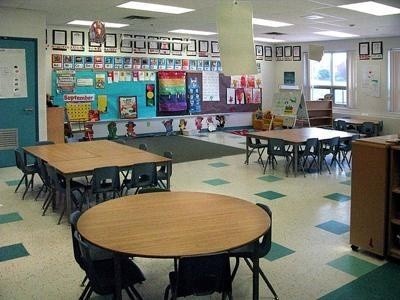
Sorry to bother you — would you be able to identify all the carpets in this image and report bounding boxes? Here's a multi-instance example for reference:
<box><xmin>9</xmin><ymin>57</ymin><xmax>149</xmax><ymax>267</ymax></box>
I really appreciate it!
<box><xmin>316</xmin><ymin>263</ymin><xmax>400</xmax><ymax>300</ymax></box>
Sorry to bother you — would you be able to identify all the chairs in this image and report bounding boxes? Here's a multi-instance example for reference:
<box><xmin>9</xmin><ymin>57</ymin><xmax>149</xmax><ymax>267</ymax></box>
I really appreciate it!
<box><xmin>243</xmin><ymin>117</ymin><xmax>384</xmax><ymax>177</ymax></box>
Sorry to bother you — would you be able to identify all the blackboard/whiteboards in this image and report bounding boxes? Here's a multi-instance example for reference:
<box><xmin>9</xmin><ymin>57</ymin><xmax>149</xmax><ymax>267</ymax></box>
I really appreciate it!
<box><xmin>51</xmin><ymin>71</ymin><xmax>263</xmax><ymax>124</ymax></box>
<box><xmin>272</xmin><ymin>91</ymin><xmax>302</xmax><ymax>116</ymax></box>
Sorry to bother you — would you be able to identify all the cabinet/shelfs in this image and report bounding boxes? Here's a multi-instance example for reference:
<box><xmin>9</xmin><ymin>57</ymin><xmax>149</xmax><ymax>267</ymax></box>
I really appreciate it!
<box><xmin>349</xmin><ymin>134</ymin><xmax>400</xmax><ymax>263</ymax></box>
<box><xmin>47</xmin><ymin>106</ymin><xmax>66</xmax><ymax>145</ymax></box>
<box><xmin>301</xmin><ymin>100</ymin><xmax>333</xmax><ymax>129</ymax></box>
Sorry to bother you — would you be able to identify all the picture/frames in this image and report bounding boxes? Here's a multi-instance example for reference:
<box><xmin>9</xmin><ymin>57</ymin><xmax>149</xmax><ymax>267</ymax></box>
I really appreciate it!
<box><xmin>372</xmin><ymin>41</ymin><xmax>382</xmax><ymax>54</ymax></box>
<box><xmin>51</xmin><ymin>29</ymin><xmax>302</xmax><ymax>57</ymax></box>
<box><xmin>119</xmin><ymin>95</ymin><xmax>138</xmax><ymax>119</ymax></box>
<box><xmin>358</xmin><ymin>42</ymin><xmax>369</xmax><ymax>55</ymax></box>
